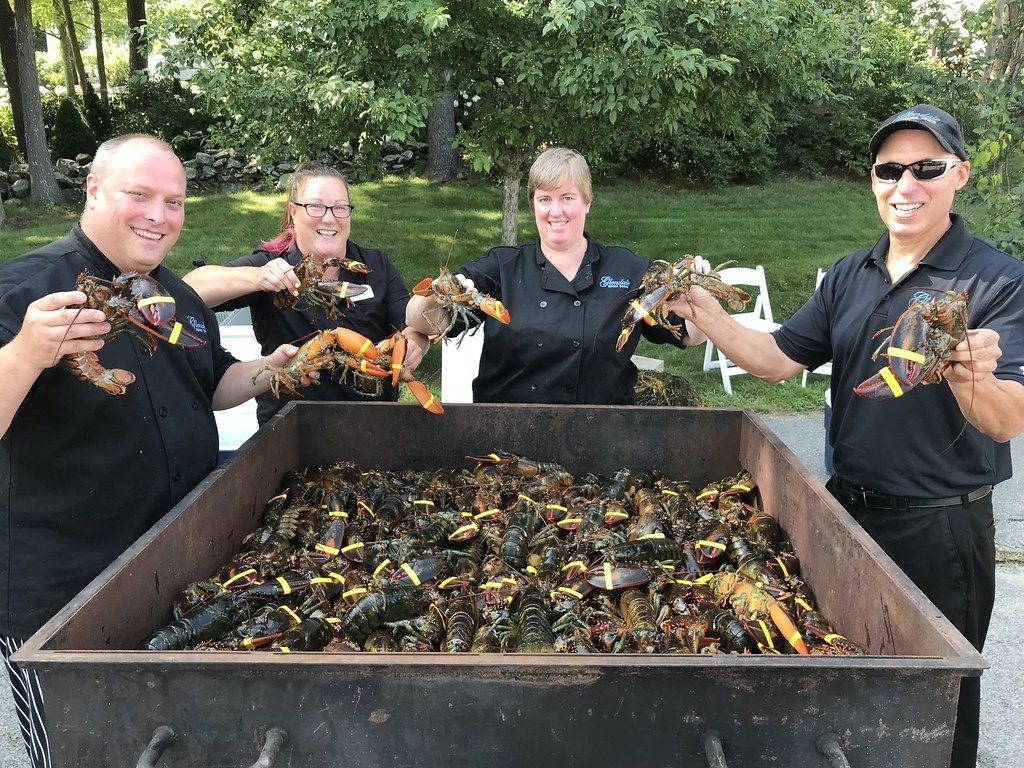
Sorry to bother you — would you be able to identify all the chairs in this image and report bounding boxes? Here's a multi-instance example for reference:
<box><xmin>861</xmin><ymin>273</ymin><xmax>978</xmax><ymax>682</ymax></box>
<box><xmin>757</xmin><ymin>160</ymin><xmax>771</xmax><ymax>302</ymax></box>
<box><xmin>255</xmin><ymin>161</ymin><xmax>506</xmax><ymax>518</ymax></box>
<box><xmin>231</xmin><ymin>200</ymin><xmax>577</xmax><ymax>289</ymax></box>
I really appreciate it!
<box><xmin>801</xmin><ymin>268</ymin><xmax>832</xmax><ymax>388</ymax></box>
<box><xmin>702</xmin><ymin>265</ymin><xmax>786</xmax><ymax>395</ymax></box>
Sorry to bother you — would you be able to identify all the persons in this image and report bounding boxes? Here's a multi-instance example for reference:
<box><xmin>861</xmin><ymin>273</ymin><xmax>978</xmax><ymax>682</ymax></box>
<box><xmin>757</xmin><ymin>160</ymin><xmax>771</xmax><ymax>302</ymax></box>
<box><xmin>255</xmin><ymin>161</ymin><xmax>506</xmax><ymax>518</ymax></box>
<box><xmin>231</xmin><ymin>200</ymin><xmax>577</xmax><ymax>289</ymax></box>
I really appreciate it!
<box><xmin>0</xmin><ymin>133</ymin><xmax>333</xmax><ymax>768</ymax></box>
<box><xmin>405</xmin><ymin>147</ymin><xmax>719</xmax><ymax>406</ymax></box>
<box><xmin>657</xmin><ymin>103</ymin><xmax>1024</xmax><ymax>768</ymax></box>
<box><xmin>181</xmin><ymin>160</ymin><xmax>429</xmax><ymax>430</ymax></box>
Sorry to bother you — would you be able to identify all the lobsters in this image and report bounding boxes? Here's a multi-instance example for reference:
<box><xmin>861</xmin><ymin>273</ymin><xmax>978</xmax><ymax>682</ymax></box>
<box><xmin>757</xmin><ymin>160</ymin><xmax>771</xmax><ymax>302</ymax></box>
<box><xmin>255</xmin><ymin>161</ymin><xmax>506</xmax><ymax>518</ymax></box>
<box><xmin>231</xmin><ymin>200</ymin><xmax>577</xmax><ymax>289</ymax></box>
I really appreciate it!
<box><xmin>60</xmin><ymin>268</ymin><xmax>210</xmax><ymax>397</ymax></box>
<box><xmin>251</xmin><ymin>327</ymin><xmax>444</xmax><ymax>415</ymax></box>
<box><xmin>844</xmin><ymin>287</ymin><xmax>975</xmax><ymax>456</ymax></box>
<box><xmin>411</xmin><ymin>228</ymin><xmax>511</xmax><ymax>349</ymax></box>
<box><xmin>270</xmin><ymin>253</ymin><xmax>373</xmax><ymax>324</ymax></box>
<box><xmin>142</xmin><ymin>446</ymin><xmax>866</xmax><ymax>655</ymax></box>
<box><xmin>616</xmin><ymin>254</ymin><xmax>752</xmax><ymax>355</ymax></box>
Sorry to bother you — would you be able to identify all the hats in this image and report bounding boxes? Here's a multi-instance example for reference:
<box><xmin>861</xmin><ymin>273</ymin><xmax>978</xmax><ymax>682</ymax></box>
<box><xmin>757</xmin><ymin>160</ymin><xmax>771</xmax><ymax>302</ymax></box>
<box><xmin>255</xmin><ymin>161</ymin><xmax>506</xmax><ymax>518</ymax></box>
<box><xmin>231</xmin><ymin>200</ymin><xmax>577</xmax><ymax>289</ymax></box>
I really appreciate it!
<box><xmin>870</xmin><ymin>104</ymin><xmax>966</xmax><ymax>160</ymax></box>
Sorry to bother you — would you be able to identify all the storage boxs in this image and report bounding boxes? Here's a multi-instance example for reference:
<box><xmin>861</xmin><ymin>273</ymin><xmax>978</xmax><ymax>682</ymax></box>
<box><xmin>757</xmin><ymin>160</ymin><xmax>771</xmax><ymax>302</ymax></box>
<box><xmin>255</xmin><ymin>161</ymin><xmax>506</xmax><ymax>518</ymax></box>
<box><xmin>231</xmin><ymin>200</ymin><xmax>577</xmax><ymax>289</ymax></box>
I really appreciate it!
<box><xmin>8</xmin><ymin>402</ymin><xmax>991</xmax><ymax>768</ymax></box>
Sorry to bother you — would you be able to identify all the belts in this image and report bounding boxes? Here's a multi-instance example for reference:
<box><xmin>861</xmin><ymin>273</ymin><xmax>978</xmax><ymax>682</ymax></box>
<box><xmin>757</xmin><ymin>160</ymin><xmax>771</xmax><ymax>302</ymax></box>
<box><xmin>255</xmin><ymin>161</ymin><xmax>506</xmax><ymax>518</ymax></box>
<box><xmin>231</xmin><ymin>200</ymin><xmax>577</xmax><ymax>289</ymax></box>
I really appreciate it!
<box><xmin>835</xmin><ymin>476</ymin><xmax>992</xmax><ymax>510</ymax></box>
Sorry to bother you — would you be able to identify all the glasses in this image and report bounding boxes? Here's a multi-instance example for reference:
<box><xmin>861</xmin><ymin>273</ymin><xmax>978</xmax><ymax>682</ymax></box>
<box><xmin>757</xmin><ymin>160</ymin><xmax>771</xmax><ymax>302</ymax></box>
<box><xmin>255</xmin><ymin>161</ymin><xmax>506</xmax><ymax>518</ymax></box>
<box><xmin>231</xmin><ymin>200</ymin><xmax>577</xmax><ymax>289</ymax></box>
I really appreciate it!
<box><xmin>873</xmin><ymin>158</ymin><xmax>961</xmax><ymax>182</ymax></box>
<box><xmin>291</xmin><ymin>201</ymin><xmax>354</xmax><ymax>218</ymax></box>
